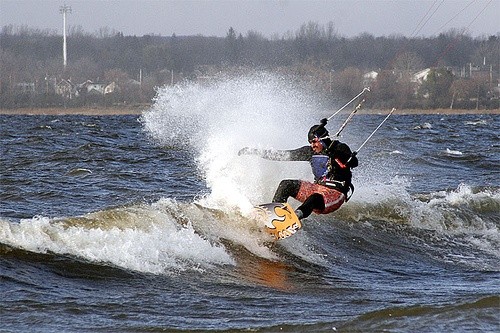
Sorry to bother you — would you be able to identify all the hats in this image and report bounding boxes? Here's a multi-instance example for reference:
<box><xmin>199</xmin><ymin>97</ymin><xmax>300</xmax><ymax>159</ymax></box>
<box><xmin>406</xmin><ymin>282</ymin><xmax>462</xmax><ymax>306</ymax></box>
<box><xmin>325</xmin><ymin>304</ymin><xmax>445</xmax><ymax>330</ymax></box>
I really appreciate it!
<box><xmin>308</xmin><ymin>125</ymin><xmax>332</xmax><ymax>148</ymax></box>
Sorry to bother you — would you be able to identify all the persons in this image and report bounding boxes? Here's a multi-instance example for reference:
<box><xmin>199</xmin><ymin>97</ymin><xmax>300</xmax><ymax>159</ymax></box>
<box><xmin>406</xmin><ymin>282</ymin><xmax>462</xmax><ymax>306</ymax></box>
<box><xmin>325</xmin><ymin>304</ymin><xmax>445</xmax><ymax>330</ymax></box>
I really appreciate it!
<box><xmin>238</xmin><ymin>125</ymin><xmax>359</xmax><ymax>220</ymax></box>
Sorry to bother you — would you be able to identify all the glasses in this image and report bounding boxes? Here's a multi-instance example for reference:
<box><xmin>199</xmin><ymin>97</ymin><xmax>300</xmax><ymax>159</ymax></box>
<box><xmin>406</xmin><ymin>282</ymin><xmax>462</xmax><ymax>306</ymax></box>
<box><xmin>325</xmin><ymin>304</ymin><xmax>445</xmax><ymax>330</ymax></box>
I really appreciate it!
<box><xmin>308</xmin><ymin>131</ymin><xmax>327</xmax><ymax>144</ymax></box>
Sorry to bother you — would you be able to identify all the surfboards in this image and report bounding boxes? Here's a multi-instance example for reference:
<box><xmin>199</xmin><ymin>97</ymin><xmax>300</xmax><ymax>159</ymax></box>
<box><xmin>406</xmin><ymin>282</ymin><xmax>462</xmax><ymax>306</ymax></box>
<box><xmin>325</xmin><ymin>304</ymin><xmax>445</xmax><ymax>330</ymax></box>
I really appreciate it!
<box><xmin>254</xmin><ymin>203</ymin><xmax>302</xmax><ymax>240</ymax></box>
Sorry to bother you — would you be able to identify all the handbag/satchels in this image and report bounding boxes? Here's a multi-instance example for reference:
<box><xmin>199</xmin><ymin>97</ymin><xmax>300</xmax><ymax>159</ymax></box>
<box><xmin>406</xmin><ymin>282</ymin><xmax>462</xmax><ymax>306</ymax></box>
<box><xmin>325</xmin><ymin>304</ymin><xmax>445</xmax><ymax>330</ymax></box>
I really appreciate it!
<box><xmin>342</xmin><ymin>181</ymin><xmax>354</xmax><ymax>202</ymax></box>
